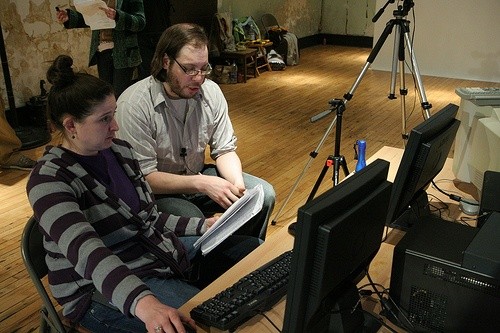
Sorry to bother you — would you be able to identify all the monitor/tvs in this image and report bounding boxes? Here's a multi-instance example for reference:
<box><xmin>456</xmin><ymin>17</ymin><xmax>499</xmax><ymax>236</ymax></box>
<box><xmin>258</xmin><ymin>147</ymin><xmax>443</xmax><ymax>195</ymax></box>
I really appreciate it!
<box><xmin>283</xmin><ymin>158</ymin><xmax>394</xmax><ymax>333</ymax></box>
<box><xmin>387</xmin><ymin>103</ymin><xmax>462</xmax><ymax>231</ymax></box>
<box><xmin>452</xmin><ymin>97</ymin><xmax>500</xmax><ymax>191</ymax></box>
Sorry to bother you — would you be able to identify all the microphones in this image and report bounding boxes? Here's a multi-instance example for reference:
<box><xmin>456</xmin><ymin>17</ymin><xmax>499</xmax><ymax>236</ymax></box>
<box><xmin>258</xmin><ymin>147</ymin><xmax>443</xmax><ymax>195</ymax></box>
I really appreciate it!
<box><xmin>180</xmin><ymin>148</ymin><xmax>187</xmax><ymax>161</ymax></box>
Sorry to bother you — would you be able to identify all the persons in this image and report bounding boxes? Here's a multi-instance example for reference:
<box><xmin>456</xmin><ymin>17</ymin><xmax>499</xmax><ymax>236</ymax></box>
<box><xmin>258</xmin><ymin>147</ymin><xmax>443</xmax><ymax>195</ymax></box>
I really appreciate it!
<box><xmin>0</xmin><ymin>93</ymin><xmax>37</xmax><ymax>170</ymax></box>
<box><xmin>26</xmin><ymin>55</ymin><xmax>265</xmax><ymax>333</ymax></box>
<box><xmin>112</xmin><ymin>24</ymin><xmax>275</xmax><ymax>240</ymax></box>
<box><xmin>56</xmin><ymin>0</ymin><xmax>146</xmax><ymax>100</ymax></box>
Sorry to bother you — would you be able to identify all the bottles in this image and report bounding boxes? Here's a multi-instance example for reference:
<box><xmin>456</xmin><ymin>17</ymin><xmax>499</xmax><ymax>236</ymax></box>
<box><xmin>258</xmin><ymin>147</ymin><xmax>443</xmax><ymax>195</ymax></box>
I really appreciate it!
<box><xmin>354</xmin><ymin>140</ymin><xmax>367</xmax><ymax>175</ymax></box>
<box><xmin>249</xmin><ymin>25</ymin><xmax>255</xmax><ymax>41</ymax></box>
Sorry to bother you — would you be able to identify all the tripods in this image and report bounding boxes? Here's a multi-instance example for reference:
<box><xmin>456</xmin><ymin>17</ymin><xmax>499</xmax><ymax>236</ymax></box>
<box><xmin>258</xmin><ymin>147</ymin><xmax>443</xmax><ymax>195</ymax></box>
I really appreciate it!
<box><xmin>271</xmin><ymin>0</ymin><xmax>431</xmax><ymax>226</ymax></box>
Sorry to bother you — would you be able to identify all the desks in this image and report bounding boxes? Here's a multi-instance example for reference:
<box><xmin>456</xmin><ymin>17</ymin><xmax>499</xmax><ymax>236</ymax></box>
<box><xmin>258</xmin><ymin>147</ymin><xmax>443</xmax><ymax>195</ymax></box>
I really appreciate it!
<box><xmin>153</xmin><ymin>146</ymin><xmax>480</xmax><ymax>333</ymax></box>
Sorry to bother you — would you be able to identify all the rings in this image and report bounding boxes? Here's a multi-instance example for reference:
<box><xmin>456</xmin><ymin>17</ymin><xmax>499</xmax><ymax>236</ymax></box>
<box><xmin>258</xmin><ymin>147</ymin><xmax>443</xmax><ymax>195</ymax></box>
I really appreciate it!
<box><xmin>155</xmin><ymin>327</ymin><xmax>162</xmax><ymax>333</ymax></box>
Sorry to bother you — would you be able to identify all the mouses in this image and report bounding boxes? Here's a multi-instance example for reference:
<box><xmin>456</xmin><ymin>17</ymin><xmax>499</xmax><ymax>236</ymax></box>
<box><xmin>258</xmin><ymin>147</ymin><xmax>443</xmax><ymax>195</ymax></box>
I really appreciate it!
<box><xmin>171</xmin><ymin>322</ymin><xmax>197</xmax><ymax>333</ymax></box>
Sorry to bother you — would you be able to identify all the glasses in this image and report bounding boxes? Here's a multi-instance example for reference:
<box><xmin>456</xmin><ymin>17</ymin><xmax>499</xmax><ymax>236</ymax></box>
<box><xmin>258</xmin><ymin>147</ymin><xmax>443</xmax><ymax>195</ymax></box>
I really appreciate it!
<box><xmin>174</xmin><ymin>57</ymin><xmax>213</xmax><ymax>76</ymax></box>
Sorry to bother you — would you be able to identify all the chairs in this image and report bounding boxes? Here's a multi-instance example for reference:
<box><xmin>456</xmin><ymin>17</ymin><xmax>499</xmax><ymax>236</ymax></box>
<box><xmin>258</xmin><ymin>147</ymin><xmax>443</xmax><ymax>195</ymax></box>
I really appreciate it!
<box><xmin>213</xmin><ymin>12</ymin><xmax>258</xmax><ymax>83</ymax></box>
<box><xmin>262</xmin><ymin>14</ymin><xmax>300</xmax><ymax>65</ymax></box>
<box><xmin>234</xmin><ymin>17</ymin><xmax>273</xmax><ymax>75</ymax></box>
<box><xmin>21</xmin><ymin>214</ymin><xmax>90</xmax><ymax>333</ymax></box>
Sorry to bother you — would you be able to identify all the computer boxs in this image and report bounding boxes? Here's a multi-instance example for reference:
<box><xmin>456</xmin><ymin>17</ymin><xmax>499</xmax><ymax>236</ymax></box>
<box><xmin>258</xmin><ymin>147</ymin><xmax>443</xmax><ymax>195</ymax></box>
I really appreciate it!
<box><xmin>384</xmin><ymin>214</ymin><xmax>500</xmax><ymax>333</ymax></box>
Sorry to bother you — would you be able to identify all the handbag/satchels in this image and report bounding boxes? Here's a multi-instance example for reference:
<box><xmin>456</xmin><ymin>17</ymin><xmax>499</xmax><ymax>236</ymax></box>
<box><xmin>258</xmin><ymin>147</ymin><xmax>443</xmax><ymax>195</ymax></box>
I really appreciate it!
<box><xmin>224</xmin><ymin>35</ymin><xmax>237</xmax><ymax>51</ymax></box>
<box><xmin>213</xmin><ymin>63</ymin><xmax>237</xmax><ymax>84</ymax></box>
<box><xmin>5</xmin><ymin>103</ymin><xmax>52</xmax><ymax>153</ymax></box>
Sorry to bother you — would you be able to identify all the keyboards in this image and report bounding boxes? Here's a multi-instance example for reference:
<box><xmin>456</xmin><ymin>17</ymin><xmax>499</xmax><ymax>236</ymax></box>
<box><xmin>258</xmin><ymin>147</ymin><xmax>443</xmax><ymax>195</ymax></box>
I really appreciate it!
<box><xmin>455</xmin><ymin>87</ymin><xmax>500</xmax><ymax>106</ymax></box>
<box><xmin>190</xmin><ymin>248</ymin><xmax>294</xmax><ymax>333</ymax></box>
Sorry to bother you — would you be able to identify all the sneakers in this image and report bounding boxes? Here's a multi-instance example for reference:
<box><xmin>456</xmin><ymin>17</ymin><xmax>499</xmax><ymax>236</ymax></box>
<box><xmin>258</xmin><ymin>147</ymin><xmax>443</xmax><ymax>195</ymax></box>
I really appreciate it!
<box><xmin>2</xmin><ymin>152</ymin><xmax>38</xmax><ymax>170</ymax></box>
